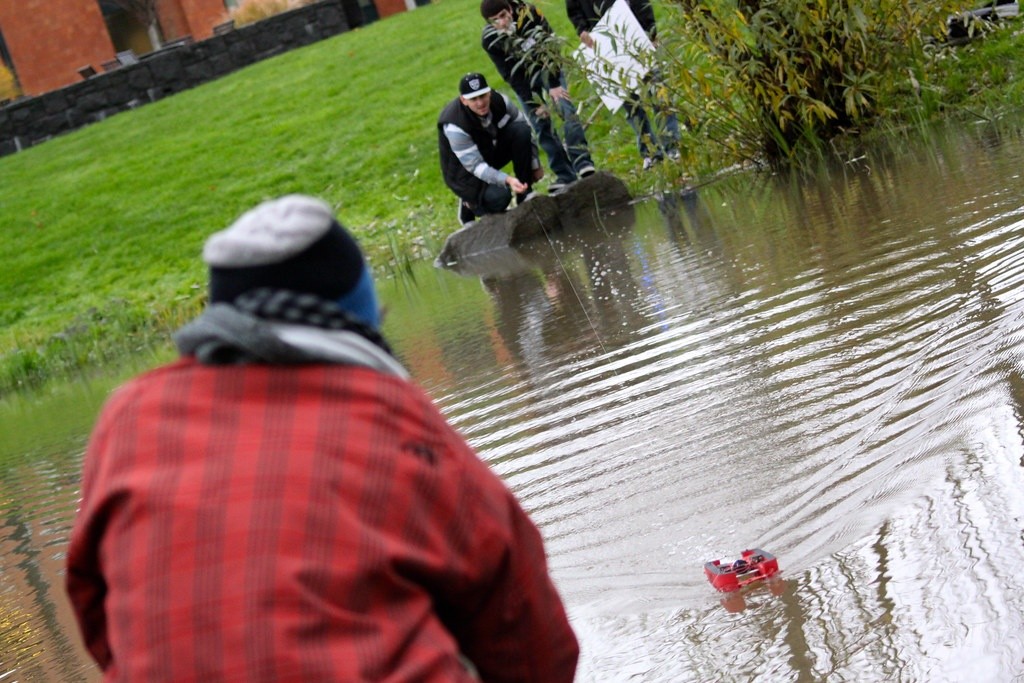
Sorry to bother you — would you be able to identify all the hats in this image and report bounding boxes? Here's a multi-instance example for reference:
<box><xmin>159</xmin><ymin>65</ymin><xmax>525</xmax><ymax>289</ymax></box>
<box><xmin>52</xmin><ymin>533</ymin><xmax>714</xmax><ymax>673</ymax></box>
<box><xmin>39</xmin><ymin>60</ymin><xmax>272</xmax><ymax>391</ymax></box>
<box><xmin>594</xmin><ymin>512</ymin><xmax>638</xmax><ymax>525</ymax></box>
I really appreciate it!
<box><xmin>202</xmin><ymin>194</ymin><xmax>379</xmax><ymax>332</ymax></box>
<box><xmin>460</xmin><ymin>73</ymin><xmax>491</xmax><ymax>100</ymax></box>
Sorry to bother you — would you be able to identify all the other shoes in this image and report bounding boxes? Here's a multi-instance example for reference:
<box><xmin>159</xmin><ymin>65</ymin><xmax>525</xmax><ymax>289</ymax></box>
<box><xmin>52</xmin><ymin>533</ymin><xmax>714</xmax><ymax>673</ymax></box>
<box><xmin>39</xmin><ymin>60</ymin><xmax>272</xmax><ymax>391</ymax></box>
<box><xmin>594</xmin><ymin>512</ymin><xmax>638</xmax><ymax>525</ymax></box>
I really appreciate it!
<box><xmin>643</xmin><ymin>158</ymin><xmax>663</xmax><ymax>171</ymax></box>
<box><xmin>577</xmin><ymin>160</ymin><xmax>596</xmax><ymax>178</ymax></box>
<box><xmin>547</xmin><ymin>175</ymin><xmax>579</xmax><ymax>194</ymax></box>
<box><xmin>457</xmin><ymin>198</ymin><xmax>476</xmax><ymax>227</ymax></box>
<box><xmin>667</xmin><ymin>149</ymin><xmax>681</xmax><ymax>159</ymax></box>
<box><xmin>516</xmin><ymin>188</ymin><xmax>538</xmax><ymax>207</ymax></box>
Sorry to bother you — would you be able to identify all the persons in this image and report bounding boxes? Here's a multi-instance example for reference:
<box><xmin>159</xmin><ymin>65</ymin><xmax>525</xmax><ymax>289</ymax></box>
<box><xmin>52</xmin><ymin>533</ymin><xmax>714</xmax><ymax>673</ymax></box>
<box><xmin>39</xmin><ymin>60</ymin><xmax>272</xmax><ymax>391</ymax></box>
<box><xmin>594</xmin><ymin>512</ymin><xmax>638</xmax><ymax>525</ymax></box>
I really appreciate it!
<box><xmin>437</xmin><ymin>72</ymin><xmax>544</xmax><ymax>226</ymax></box>
<box><xmin>481</xmin><ymin>0</ymin><xmax>597</xmax><ymax>191</ymax></box>
<box><xmin>64</xmin><ymin>193</ymin><xmax>578</xmax><ymax>683</ymax></box>
<box><xmin>565</xmin><ymin>0</ymin><xmax>683</xmax><ymax>174</ymax></box>
